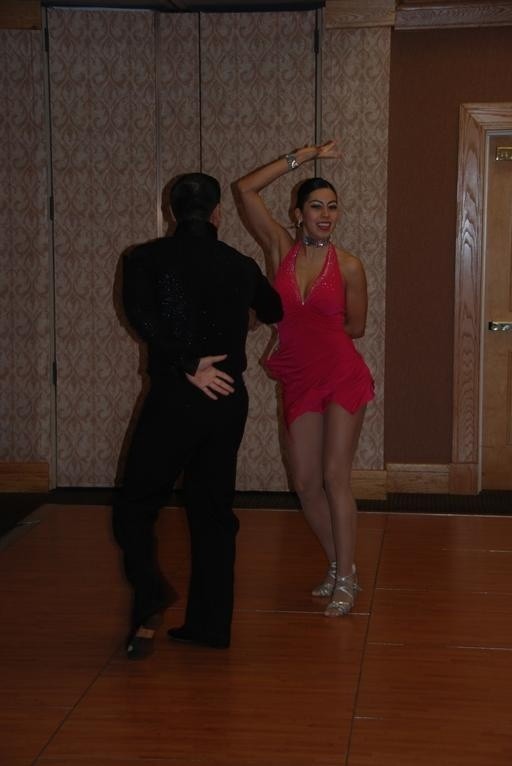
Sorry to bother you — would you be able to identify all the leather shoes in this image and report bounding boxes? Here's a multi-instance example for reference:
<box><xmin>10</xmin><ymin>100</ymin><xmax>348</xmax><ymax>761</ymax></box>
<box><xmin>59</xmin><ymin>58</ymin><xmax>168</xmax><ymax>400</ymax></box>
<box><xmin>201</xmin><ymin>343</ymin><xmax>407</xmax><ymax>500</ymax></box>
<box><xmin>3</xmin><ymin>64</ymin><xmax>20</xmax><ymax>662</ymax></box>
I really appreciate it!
<box><xmin>167</xmin><ymin>622</ymin><xmax>231</xmax><ymax>649</ymax></box>
<box><xmin>125</xmin><ymin>588</ymin><xmax>179</xmax><ymax>662</ymax></box>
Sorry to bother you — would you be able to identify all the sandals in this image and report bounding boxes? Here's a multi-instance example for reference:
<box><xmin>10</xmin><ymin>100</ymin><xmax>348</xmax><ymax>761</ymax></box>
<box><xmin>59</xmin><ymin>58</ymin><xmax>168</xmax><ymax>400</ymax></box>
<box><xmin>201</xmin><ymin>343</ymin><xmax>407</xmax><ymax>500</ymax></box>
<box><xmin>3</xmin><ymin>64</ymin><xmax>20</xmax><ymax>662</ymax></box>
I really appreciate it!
<box><xmin>323</xmin><ymin>562</ymin><xmax>362</xmax><ymax>618</ymax></box>
<box><xmin>312</xmin><ymin>561</ymin><xmax>338</xmax><ymax>597</ymax></box>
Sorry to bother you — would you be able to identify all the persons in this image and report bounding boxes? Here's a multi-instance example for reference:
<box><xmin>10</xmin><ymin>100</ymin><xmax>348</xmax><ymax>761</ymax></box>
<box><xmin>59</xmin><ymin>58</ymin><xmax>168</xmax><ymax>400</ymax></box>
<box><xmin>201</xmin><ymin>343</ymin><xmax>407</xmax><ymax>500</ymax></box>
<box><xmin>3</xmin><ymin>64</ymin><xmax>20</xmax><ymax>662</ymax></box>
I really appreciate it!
<box><xmin>108</xmin><ymin>169</ymin><xmax>284</xmax><ymax>659</ymax></box>
<box><xmin>236</xmin><ymin>142</ymin><xmax>379</xmax><ymax>620</ymax></box>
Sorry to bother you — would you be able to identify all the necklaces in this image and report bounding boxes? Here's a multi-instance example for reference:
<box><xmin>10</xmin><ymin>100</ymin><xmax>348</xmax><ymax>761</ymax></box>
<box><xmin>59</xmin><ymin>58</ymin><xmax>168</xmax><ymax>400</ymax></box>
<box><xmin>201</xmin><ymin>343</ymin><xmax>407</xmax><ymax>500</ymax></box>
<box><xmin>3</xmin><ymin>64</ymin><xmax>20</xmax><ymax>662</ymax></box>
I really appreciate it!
<box><xmin>301</xmin><ymin>234</ymin><xmax>331</xmax><ymax>248</ymax></box>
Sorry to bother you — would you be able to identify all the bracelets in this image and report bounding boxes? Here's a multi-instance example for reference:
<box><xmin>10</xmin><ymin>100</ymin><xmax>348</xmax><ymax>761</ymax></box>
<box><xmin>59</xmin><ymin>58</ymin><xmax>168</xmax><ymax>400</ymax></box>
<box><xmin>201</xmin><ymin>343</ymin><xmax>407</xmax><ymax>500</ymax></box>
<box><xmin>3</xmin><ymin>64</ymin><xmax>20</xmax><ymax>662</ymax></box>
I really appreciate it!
<box><xmin>279</xmin><ymin>152</ymin><xmax>299</xmax><ymax>170</ymax></box>
